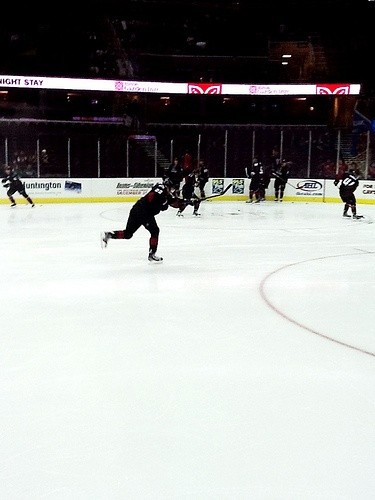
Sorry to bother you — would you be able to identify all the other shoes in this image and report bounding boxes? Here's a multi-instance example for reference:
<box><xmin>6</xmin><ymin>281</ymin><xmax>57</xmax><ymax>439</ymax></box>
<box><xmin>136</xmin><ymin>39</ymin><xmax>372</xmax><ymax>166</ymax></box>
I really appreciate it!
<box><xmin>353</xmin><ymin>213</ymin><xmax>363</xmax><ymax>220</ymax></box>
<box><xmin>11</xmin><ymin>204</ymin><xmax>16</xmax><ymax>207</ymax></box>
<box><xmin>246</xmin><ymin>200</ymin><xmax>253</xmax><ymax>203</ymax></box>
<box><xmin>280</xmin><ymin>198</ymin><xmax>283</xmax><ymax>202</ymax></box>
<box><xmin>274</xmin><ymin>198</ymin><xmax>278</xmax><ymax>201</ymax></box>
<box><xmin>342</xmin><ymin>213</ymin><xmax>351</xmax><ymax>217</ymax></box>
<box><xmin>31</xmin><ymin>204</ymin><xmax>35</xmax><ymax>208</ymax></box>
<box><xmin>254</xmin><ymin>199</ymin><xmax>262</xmax><ymax>204</ymax></box>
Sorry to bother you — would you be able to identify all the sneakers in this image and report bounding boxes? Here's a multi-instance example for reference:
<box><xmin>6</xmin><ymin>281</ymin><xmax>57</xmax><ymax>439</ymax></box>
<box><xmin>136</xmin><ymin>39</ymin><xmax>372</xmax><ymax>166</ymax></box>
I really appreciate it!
<box><xmin>192</xmin><ymin>210</ymin><xmax>201</xmax><ymax>216</ymax></box>
<box><xmin>101</xmin><ymin>231</ymin><xmax>110</xmax><ymax>247</ymax></box>
<box><xmin>148</xmin><ymin>254</ymin><xmax>163</xmax><ymax>263</ymax></box>
<box><xmin>176</xmin><ymin>211</ymin><xmax>184</xmax><ymax>218</ymax></box>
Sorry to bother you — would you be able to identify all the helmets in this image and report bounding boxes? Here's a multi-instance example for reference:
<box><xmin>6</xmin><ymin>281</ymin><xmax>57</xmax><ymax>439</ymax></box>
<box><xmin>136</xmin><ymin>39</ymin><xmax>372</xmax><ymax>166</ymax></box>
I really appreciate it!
<box><xmin>5</xmin><ymin>166</ymin><xmax>11</xmax><ymax>175</ymax></box>
<box><xmin>193</xmin><ymin>169</ymin><xmax>200</xmax><ymax>175</ymax></box>
<box><xmin>253</xmin><ymin>159</ymin><xmax>259</xmax><ymax>167</ymax></box>
<box><xmin>281</xmin><ymin>159</ymin><xmax>287</xmax><ymax>166</ymax></box>
<box><xmin>164</xmin><ymin>178</ymin><xmax>174</xmax><ymax>188</ymax></box>
<box><xmin>352</xmin><ymin>170</ymin><xmax>358</xmax><ymax>178</ymax></box>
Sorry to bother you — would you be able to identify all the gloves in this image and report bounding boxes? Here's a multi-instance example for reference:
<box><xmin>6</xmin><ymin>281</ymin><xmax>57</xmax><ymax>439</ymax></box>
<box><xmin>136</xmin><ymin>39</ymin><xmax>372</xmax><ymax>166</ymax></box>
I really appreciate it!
<box><xmin>334</xmin><ymin>181</ymin><xmax>339</xmax><ymax>186</ymax></box>
<box><xmin>190</xmin><ymin>198</ymin><xmax>198</xmax><ymax>206</ymax></box>
<box><xmin>1</xmin><ymin>179</ymin><xmax>6</xmax><ymax>183</ymax></box>
<box><xmin>3</xmin><ymin>183</ymin><xmax>9</xmax><ymax>188</ymax></box>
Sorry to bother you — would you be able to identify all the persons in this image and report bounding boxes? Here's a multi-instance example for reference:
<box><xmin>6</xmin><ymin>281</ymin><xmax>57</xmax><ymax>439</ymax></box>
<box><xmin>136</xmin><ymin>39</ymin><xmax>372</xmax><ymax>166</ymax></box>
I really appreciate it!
<box><xmin>163</xmin><ymin>145</ymin><xmax>292</xmax><ymax>218</ymax></box>
<box><xmin>0</xmin><ymin>145</ymin><xmax>50</xmax><ymax>208</ymax></box>
<box><xmin>101</xmin><ymin>178</ymin><xmax>198</xmax><ymax>264</ymax></box>
<box><xmin>318</xmin><ymin>160</ymin><xmax>375</xmax><ymax>219</ymax></box>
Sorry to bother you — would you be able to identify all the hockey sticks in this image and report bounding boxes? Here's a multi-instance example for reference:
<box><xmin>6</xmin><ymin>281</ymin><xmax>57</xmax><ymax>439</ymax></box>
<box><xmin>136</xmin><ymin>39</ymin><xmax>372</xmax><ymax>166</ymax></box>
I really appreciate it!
<box><xmin>279</xmin><ymin>176</ymin><xmax>304</xmax><ymax>189</ymax></box>
<box><xmin>196</xmin><ymin>183</ymin><xmax>233</xmax><ymax>200</ymax></box>
